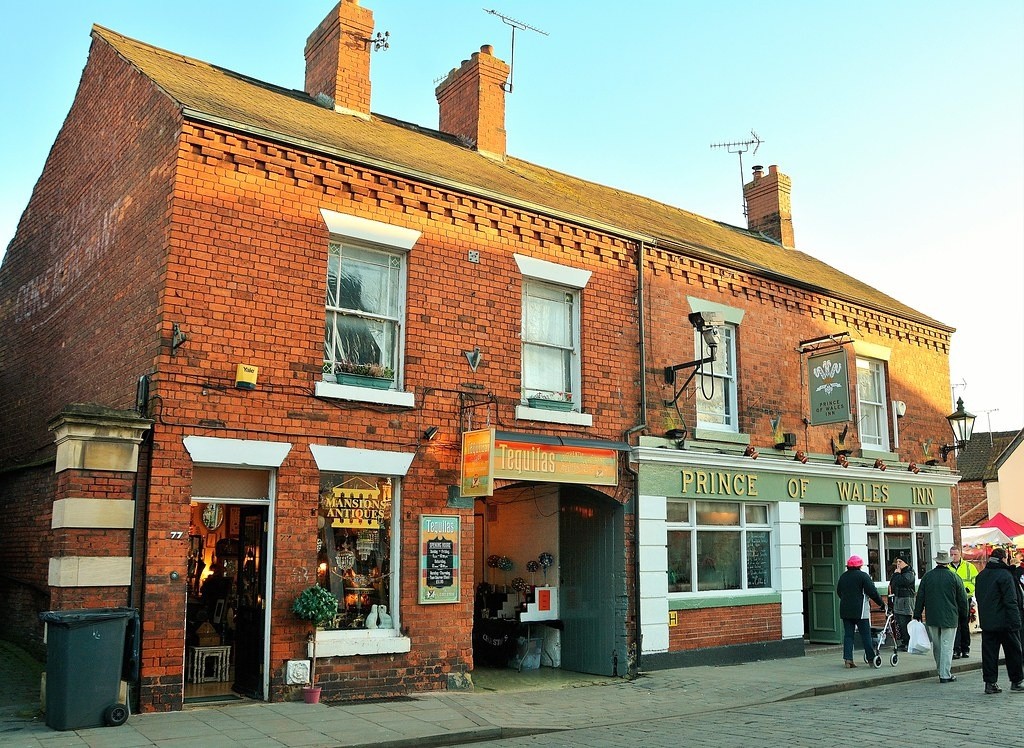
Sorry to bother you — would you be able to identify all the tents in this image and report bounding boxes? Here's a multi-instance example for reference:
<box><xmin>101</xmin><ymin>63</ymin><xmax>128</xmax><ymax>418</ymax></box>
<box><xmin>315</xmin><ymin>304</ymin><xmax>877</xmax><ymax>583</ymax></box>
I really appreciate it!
<box><xmin>961</xmin><ymin>528</ymin><xmax>1012</xmax><ymax>559</ymax></box>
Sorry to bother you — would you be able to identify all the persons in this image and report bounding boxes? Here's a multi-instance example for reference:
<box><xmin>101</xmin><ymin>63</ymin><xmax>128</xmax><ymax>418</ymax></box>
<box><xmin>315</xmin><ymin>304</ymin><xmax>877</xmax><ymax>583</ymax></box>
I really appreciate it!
<box><xmin>888</xmin><ymin>557</ymin><xmax>915</xmax><ymax>653</ymax></box>
<box><xmin>913</xmin><ymin>550</ymin><xmax>969</xmax><ymax>683</ymax></box>
<box><xmin>975</xmin><ymin>548</ymin><xmax>1024</xmax><ymax>693</ymax></box>
<box><xmin>945</xmin><ymin>547</ymin><xmax>979</xmax><ymax>659</ymax></box>
<box><xmin>836</xmin><ymin>556</ymin><xmax>887</xmax><ymax>668</ymax></box>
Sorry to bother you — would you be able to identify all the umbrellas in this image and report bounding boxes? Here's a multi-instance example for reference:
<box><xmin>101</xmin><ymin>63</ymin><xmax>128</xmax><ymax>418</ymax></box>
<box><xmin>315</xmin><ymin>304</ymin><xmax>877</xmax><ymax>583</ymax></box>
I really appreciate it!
<box><xmin>981</xmin><ymin>513</ymin><xmax>1024</xmax><ymax>538</ymax></box>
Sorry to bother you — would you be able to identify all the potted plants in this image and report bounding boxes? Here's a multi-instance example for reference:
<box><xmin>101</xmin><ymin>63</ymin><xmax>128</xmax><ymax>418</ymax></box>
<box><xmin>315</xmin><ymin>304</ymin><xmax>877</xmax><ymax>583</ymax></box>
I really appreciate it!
<box><xmin>293</xmin><ymin>583</ymin><xmax>337</xmax><ymax>705</ymax></box>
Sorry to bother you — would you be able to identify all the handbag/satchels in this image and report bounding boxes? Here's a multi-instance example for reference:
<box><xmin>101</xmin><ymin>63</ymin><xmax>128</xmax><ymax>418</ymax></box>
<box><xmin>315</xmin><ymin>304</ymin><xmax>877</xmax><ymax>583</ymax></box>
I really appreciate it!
<box><xmin>970</xmin><ymin>596</ymin><xmax>978</xmax><ymax>623</ymax></box>
<box><xmin>907</xmin><ymin>619</ymin><xmax>931</xmax><ymax>655</ymax></box>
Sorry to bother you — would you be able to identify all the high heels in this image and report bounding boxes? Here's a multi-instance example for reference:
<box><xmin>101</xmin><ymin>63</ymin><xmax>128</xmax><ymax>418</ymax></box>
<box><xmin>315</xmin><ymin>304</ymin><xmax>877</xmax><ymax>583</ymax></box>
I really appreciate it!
<box><xmin>868</xmin><ymin>660</ymin><xmax>874</xmax><ymax>668</ymax></box>
<box><xmin>845</xmin><ymin>660</ymin><xmax>857</xmax><ymax>668</ymax></box>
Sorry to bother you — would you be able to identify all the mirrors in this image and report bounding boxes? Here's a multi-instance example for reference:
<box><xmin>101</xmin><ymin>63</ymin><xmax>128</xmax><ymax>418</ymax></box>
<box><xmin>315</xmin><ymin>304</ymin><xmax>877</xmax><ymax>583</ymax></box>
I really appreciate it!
<box><xmin>200</xmin><ymin>503</ymin><xmax>224</xmax><ymax>532</ymax></box>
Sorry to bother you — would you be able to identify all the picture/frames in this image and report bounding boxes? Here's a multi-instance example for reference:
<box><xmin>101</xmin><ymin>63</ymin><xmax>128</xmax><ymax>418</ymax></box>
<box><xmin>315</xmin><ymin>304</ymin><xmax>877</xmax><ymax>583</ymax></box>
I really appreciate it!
<box><xmin>225</xmin><ymin>505</ymin><xmax>240</xmax><ymax>539</ymax></box>
<box><xmin>417</xmin><ymin>514</ymin><xmax>461</xmax><ymax>605</ymax></box>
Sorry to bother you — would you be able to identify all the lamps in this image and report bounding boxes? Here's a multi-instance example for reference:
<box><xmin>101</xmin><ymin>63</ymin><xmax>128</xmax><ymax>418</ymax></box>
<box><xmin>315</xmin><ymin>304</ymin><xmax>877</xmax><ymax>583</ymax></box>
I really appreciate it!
<box><xmin>896</xmin><ymin>514</ymin><xmax>903</xmax><ymax>525</ymax></box>
<box><xmin>796</xmin><ymin>450</ymin><xmax>809</xmax><ymax>464</ymax></box>
<box><xmin>838</xmin><ymin>455</ymin><xmax>849</xmax><ymax>468</ymax></box>
<box><xmin>910</xmin><ymin>461</ymin><xmax>919</xmax><ymax>475</ymax></box>
<box><xmin>234</xmin><ymin>363</ymin><xmax>258</xmax><ymax>389</ymax></box>
<box><xmin>776</xmin><ymin>433</ymin><xmax>796</xmax><ymax>450</ymax></box>
<box><xmin>888</xmin><ymin>515</ymin><xmax>894</xmax><ymax>526</ymax></box>
<box><xmin>941</xmin><ymin>397</ymin><xmax>978</xmax><ymax>463</ymax></box>
<box><xmin>665</xmin><ymin>428</ymin><xmax>688</xmax><ymax>450</ymax></box>
<box><xmin>746</xmin><ymin>446</ymin><xmax>760</xmax><ymax>460</ymax></box>
<box><xmin>876</xmin><ymin>457</ymin><xmax>886</xmax><ymax>471</ymax></box>
<box><xmin>421</xmin><ymin>426</ymin><xmax>439</xmax><ymax>440</ymax></box>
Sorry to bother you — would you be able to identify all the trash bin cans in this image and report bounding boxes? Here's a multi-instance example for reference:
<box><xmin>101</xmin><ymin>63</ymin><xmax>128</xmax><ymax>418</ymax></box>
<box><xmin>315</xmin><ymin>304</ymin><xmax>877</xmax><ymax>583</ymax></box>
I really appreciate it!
<box><xmin>40</xmin><ymin>606</ymin><xmax>141</xmax><ymax>731</ymax></box>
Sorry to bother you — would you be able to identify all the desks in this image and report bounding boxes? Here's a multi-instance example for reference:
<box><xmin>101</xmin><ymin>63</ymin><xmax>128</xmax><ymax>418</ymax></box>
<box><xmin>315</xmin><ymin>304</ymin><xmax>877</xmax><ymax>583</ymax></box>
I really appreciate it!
<box><xmin>477</xmin><ymin>616</ymin><xmax>565</xmax><ymax>673</ymax></box>
<box><xmin>187</xmin><ymin>645</ymin><xmax>231</xmax><ymax>683</ymax></box>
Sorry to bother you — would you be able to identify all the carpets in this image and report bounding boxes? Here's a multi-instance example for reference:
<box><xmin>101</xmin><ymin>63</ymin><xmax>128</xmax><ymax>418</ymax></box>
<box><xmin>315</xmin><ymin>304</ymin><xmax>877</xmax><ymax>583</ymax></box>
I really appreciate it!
<box><xmin>184</xmin><ymin>693</ymin><xmax>244</xmax><ymax>704</ymax></box>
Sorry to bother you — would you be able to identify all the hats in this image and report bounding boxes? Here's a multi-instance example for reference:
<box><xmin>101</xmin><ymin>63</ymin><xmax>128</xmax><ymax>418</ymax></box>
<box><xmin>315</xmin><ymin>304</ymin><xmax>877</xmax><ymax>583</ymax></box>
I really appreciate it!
<box><xmin>932</xmin><ymin>550</ymin><xmax>954</xmax><ymax>563</ymax></box>
<box><xmin>896</xmin><ymin>555</ymin><xmax>909</xmax><ymax>564</ymax></box>
<box><xmin>847</xmin><ymin>555</ymin><xmax>864</xmax><ymax>567</ymax></box>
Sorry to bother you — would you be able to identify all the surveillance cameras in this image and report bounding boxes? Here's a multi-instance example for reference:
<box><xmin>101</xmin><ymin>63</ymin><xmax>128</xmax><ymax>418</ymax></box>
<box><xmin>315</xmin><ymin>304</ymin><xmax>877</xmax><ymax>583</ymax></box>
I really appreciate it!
<box><xmin>688</xmin><ymin>310</ymin><xmax>725</xmax><ymax>329</ymax></box>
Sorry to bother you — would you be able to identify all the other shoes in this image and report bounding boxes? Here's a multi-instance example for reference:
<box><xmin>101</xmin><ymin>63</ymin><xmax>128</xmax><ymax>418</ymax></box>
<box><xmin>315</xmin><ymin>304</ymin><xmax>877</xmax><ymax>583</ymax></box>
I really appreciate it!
<box><xmin>962</xmin><ymin>652</ymin><xmax>970</xmax><ymax>658</ymax></box>
<box><xmin>938</xmin><ymin>675</ymin><xmax>957</xmax><ymax>683</ymax></box>
<box><xmin>897</xmin><ymin>643</ymin><xmax>908</xmax><ymax>652</ymax></box>
<box><xmin>1009</xmin><ymin>679</ymin><xmax>1024</xmax><ymax>693</ymax></box>
<box><xmin>953</xmin><ymin>651</ymin><xmax>961</xmax><ymax>658</ymax></box>
<box><xmin>985</xmin><ymin>682</ymin><xmax>1002</xmax><ymax>694</ymax></box>
